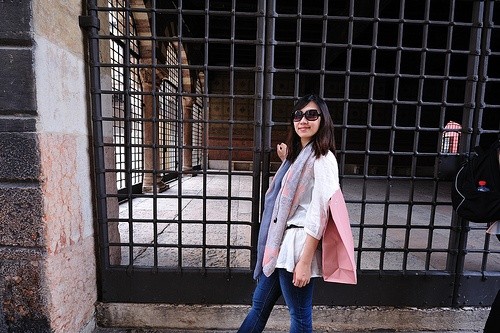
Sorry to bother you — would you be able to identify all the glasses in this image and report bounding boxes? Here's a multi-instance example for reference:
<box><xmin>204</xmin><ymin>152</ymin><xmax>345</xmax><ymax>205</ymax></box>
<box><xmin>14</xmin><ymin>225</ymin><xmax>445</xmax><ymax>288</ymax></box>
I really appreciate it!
<box><xmin>292</xmin><ymin>109</ymin><xmax>322</xmax><ymax>122</ymax></box>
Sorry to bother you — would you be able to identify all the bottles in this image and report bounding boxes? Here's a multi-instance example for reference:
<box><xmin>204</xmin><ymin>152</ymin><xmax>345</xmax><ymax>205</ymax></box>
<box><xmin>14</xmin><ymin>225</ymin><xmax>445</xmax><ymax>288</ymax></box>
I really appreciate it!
<box><xmin>476</xmin><ymin>181</ymin><xmax>489</xmax><ymax>192</ymax></box>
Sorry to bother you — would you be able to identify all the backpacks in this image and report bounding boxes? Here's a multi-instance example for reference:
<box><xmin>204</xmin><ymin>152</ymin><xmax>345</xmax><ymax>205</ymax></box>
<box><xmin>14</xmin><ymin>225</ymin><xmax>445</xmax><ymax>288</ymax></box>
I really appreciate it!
<box><xmin>449</xmin><ymin>132</ymin><xmax>500</xmax><ymax>223</ymax></box>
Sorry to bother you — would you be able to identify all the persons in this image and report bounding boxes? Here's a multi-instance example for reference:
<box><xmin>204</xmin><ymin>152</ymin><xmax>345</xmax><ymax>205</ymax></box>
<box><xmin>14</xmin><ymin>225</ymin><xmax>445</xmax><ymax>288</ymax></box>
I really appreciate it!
<box><xmin>236</xmin><ymin>92</ymin><xmax>357</xmax><ymax>333</ymax></box>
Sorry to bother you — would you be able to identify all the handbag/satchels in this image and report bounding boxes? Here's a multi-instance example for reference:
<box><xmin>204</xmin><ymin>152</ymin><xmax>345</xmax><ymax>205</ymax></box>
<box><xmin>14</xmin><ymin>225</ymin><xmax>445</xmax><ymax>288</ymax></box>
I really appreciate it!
<box><xmin>321</xmin><ymin>189</ymin><xmax>358</xmax><ymax>285</ymax></box>
